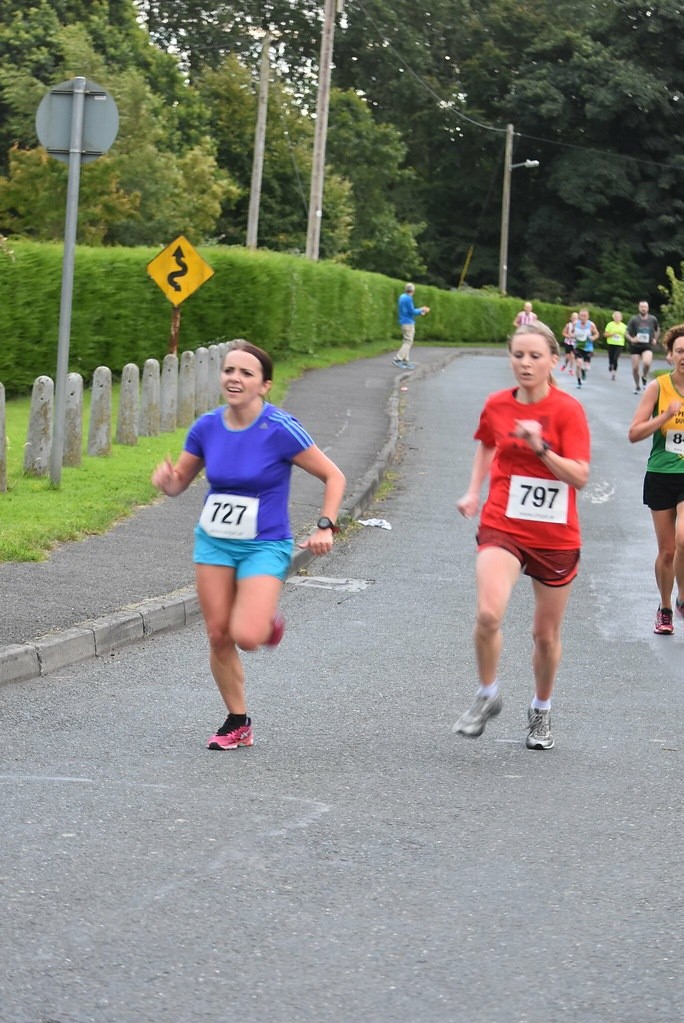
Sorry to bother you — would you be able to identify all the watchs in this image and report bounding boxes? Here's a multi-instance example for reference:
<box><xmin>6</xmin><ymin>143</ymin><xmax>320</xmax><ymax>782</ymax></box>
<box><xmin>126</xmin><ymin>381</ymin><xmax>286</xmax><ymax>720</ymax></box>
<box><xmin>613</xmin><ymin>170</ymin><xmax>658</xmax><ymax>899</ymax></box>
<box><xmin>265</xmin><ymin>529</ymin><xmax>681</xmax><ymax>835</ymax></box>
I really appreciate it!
<box><xmin>536</xmin><ymin>440</ymin><xmax>548</xmax><ymax>456</ymax></box>
<box><xmin>317</xmin><ymin>516</ymin><xmax>340</xmax><ymax>533</ymax></box>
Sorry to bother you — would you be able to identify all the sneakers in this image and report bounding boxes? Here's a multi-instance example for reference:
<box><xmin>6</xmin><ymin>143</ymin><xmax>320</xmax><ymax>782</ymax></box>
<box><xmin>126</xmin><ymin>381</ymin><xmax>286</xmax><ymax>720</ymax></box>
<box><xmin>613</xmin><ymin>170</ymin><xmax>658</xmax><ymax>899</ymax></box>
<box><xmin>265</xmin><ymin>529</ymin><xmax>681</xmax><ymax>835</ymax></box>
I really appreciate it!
<box><xmin>568</xmin><ymin>368</ymin><xmax>574</xmax><ymax>376</ymax></box>
<box><xmin>205</xmin><ymin>716</ymin><xmax>254</xmax><ymax>750</ymax></box>
<box><xmin>267</xmin><ymin>608</ymin><xmax>286</xmax><ymax>649</ymax></box>
<box><xmin>523</xmin><ymin>702</ymin><xmax>556</xmax><ymax>750</ymax></box>
<box><xmin>402</xmin><ymin>359</ymin><xmax>415</xmax><ymax>370</ymax></box>
<box><xmin>391</xmin><ymin>357</ymin><xmax>407</xmax><ymax>369</ymax></box>
<box><xmin>560</xmin><ymin>364</ymin><xmax>565</xmax><ymax>374</ymax></box>
<box><xmin>652</xmin><ymin>602</ymin><xmax>676</xmax><ymax>635</ymax></box>
<box><xmin>449</xmin><ymin>691</ymin><xmax>503</xmax><ymax>739</ymax></box>
<box><xmin>673</xmin><ymin>597</ymin><xmax>684</xmax><ymax>619</ymax></box>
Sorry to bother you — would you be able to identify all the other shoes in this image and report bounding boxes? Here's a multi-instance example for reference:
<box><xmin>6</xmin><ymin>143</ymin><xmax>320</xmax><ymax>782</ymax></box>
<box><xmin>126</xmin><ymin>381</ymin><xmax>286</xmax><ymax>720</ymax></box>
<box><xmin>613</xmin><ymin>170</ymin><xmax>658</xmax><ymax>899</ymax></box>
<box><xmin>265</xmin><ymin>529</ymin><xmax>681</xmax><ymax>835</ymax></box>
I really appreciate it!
<box><xmin>576</xmin><ymin>383</ymin><xmax>582</xmax><ymax>389</ymax></box>
<box><xmin>642</xmin><ymin>380</ymin><xmax>647</xmax><ymax>390</ymax></box>
<box><xmin>581</xmin><ymin>369</ymin><xmax>586</xmax><ymax>380</ymax></box>
<box><xmin>634</xmin><ymin>386</ymin><xmax>641</xmax><ymax>395</ymax></box>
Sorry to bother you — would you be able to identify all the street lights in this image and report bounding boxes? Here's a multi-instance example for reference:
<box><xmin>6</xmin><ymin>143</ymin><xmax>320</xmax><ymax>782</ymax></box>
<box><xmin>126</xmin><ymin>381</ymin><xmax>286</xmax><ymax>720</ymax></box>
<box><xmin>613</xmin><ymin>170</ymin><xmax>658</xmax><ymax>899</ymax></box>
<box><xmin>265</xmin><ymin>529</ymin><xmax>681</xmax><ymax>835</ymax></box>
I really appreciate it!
<box><xmin>499</xmin><ymin>160</ymin><xmax>541</xmax><ymax>294</ymax></box>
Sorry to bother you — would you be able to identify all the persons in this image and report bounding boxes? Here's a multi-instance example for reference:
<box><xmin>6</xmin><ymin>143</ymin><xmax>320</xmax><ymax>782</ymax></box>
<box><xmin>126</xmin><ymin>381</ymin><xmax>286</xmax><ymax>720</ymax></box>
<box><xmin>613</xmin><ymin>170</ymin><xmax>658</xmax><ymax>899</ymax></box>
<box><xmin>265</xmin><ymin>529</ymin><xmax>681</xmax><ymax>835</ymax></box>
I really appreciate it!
<box><xmin>603</xmin><ymin>311</ymin><xmax>627</xmax><ymax>381</ymax></box>
<box><xmin>453</xmin><ymin>319</ymin><xmax>592</xmax><ymax>750</ymax></box>
<box><xmin>628</xmin><ymin>324</ymin><xmax>684</xmax><ymax>637</ymax></box>
<box><xmin>559</xmin><ymin>308</ymin><xmax>599</xmax><ymax>389</ymax></box>
<box><xmin>624</xmin><ymin>301</ymin><xmax>660</xmax><ymax>395</ymax></box>
<box><xmin>150</xmin><ymin>342</ymin><xmax>348</xmax><ymax>751</ymax></box>
<box><xmin>392</xmin><ymin>283</ymin><xmax>431</xmax><ymax>370</ymax></box>
<box><xmin>513</xmin><ymin>301</ymin><xmax>538</xmax><ymax>330</ymax></box>
